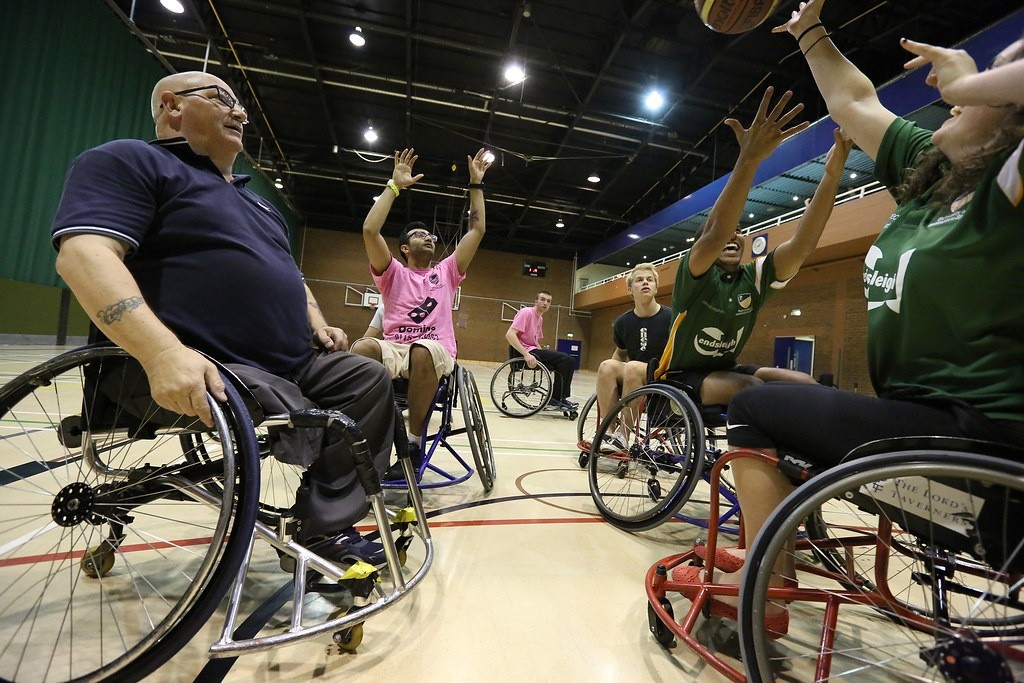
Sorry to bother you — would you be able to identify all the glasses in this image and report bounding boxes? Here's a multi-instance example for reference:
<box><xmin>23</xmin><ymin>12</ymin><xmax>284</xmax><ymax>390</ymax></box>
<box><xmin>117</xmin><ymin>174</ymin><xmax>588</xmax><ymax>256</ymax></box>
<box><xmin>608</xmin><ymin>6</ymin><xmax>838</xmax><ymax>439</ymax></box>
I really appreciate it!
<box><xmin>405</xmin><ymin>230</ymin><xmax>438</xmax><ymax>244</ymax></box>
<box><xmin>159</xmin><ymin>84</ymin><xmax>250</xmax><ymax>124</ymax></box>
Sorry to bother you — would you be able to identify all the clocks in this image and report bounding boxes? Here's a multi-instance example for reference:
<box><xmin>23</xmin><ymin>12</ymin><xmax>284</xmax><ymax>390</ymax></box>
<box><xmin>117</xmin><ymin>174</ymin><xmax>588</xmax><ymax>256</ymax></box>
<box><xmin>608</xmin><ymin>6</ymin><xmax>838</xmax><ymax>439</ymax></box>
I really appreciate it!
<box><xmin>752</xmin><ymin>237</ymin><xmax>766</xmax><ymax>254</ymax></box>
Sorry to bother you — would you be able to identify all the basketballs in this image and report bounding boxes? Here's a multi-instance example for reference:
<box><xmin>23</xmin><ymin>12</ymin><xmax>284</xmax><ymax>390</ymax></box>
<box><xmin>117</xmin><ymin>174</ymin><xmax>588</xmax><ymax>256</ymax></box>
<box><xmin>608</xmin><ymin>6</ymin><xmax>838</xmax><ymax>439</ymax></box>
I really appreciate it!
<box><xmin>692</xmin><ymin>0</ymin><xmax>778</xmax><ymax>34</ymax></box>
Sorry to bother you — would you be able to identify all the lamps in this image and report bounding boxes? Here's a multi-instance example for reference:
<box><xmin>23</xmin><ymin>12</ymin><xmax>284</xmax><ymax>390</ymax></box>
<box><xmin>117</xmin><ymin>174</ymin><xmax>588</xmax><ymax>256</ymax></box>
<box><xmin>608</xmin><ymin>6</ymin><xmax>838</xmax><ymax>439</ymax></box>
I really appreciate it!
<box><xmin>348</xmin><ymin>26</ymin><xmax>366</xmax><ymax>46</ymax></box>
<box><xmin>363</xmin><ymin>126</ymin><xmax>378</xmax><ymax>142</ymax></box>
<box><xmin>556</xmin><ymin>217</ymin><xmax>565</xmax><ymax>228</ymax></box>
<box><xmin>274</xmin><ymin>172</ymin><xmax>284</xmax><ymax>189</ymax></box>
<box><xmin>587</xmin><ymin>171</ymin><xmax>601</xmax><ymax>183</ymax></box>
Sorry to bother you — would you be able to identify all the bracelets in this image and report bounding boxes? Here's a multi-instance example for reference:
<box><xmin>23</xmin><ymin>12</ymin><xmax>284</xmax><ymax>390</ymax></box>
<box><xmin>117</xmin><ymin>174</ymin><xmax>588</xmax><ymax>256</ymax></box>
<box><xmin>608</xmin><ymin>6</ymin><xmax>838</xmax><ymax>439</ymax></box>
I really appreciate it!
<box><xmin>387</xmin><ymin>180</ymin><xmax>400</xmax><ymax>197</ymax></box>
<box><xmin>468</xmin><ymin>182</ymin><xmax>485</xmax><ymax>189</ymax></box>
<box><xmin>797</xmin><ymin>23</ymin><xmax>824</xmax><ymax>45</ymax></box>
<box><xmin>803</xmin><ymin>35</ymin><xmax>830</xmax><ymax>56</ymax></box>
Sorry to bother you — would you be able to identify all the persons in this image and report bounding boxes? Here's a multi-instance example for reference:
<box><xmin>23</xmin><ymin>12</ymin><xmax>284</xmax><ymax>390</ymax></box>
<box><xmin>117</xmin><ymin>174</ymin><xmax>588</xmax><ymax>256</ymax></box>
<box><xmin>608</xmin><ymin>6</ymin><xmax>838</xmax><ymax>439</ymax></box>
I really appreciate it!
<box><xmin>671</xmin><ymin>0</ymin><xmax>1024</xmax><ymax>640</ymax></box>
<box><xmin>350</xmin><ymin>148</ymin><xmax>512</xmax><ymax>484</ymax></box>
<box><xmin>362</xmin><ymin>303</ymin><xmax>384</xmax><ymax>338</ymax></box>
<box><xmin>586</xmin><ymin>263</ymin><xmax>672</xmax><ymax>453</ymax></box>
<box><xmin>51</xmin><ymin>71</ymin><xmax>395</xmax><ymax>573</ymax></box>
<box><xmin>653</xmin><ymin>86</ymin><xmax>854</xmax><ymax>417</ymax></box>
<box><xmin>505</xmin><ymin>290</ymin><xmax>579</xmax><ymax>410</ymax></box>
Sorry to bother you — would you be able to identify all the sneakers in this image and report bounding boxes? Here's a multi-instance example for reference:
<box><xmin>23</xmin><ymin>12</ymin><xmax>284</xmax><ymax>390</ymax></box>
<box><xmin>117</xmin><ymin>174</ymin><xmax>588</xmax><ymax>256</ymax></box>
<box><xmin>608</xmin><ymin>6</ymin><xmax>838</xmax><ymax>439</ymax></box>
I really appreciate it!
<box><xmin>575</xmin><ymin>428</ymin><xmax>613</xmax><ymax>454</ymax></box>
<box><xmin>601</xmin><ymin>432</ymin><xmax>630</xmax><ymax>452</ymax></box>
<box><xmin>545</xmin><ymin>396</ymin><xmax>579</xmax><ymax>409</ymax></box>
<box><xmin>388</xmin><ymin>442</ymin><xmax>420</xmax><ymax>478</ymax></box>
<box><xmin>280</xmin><ymin>525</ymin><xmax>406</xmax><ymax>574</ymax></box>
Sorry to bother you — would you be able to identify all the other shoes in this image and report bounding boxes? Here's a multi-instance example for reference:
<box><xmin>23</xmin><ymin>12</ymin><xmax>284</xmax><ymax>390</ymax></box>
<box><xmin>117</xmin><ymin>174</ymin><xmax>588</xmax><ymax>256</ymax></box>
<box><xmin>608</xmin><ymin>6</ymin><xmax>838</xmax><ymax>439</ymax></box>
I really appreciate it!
<box><xmin>695</xmin><ymin>545</ymin><xmax>796</xmax><ymax>604</ymax></box>
<box><xmin>672</xmin><ymin>565</ymin><xmax>789</xmax><ymax>639</ymax></box>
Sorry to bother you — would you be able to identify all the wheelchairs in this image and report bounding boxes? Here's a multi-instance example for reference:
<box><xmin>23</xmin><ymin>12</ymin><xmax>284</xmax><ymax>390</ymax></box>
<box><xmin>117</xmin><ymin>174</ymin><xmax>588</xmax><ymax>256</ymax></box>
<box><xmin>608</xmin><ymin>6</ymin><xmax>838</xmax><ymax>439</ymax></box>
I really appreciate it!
<box><xmin>577</xmin><ymin>358</ymin><xmax>808</xmax><ymax>541</ymax></box>
<box><xmin>646</xmin><ymin>435</ymin><xmax>1024</xmax><ymax>683</ymax></box>
<box><xmin>381</xmin><ymin>340</ymin><xmax>497</xmax><ymax>506</ymax></box>
<box><xmin>489</xmin><ymin>344</ymin><xmax>579</xmax><ymax>422</ymax></box>
<box><xmin>0</xmin><ymin>318</ymin><xmax>434</xmax><ymax>683</ymax></box>
